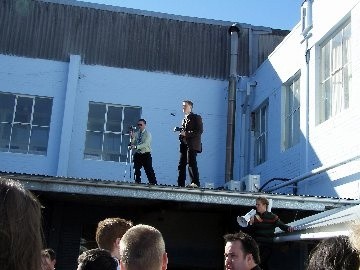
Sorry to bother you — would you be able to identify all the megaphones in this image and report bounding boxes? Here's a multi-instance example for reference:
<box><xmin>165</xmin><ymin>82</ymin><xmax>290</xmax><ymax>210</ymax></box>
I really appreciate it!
<box><xmin>237</xmin><ymin>209</ymin><xmax>256</xmax><ymax>227</ymax></box>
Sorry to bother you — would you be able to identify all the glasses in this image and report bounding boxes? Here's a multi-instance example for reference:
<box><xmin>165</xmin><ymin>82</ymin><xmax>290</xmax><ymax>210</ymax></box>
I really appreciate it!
<box><xmin>137</xmin><ymin>124</ymin><xmax>142</xmax><ymax>126</ymax></box>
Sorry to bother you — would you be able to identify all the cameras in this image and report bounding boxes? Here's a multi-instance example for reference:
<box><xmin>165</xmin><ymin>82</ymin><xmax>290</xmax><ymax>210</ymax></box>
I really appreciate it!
<box><xmin>130</xmin><ymin>125</ymin><xmax>136</xmax><ymax>131</ymax></box>
<box><xmin>174</xmin><ymin>126</ymin><xmax>184</xmax><ymax>139</ymax></box>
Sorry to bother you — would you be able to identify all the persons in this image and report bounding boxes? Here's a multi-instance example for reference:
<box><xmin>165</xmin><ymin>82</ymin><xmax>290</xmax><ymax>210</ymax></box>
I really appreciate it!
<box><xmin>249</xmin><ymin>196</ymin><xmax>297</xmax><ymax>270</ymax></box>
<box><xmin>96</xmin><ymin>217</ymin><xmax>134</xmax><ymax>270</ymax></box>
<box><xmin>305</xmin><ymin>235</ymin><xmax>360</xmax><ymax>270</ymax></box>
<box><xmin>44</xmin><ymin>249</ymin><xmax>56</xmax><ymax>270</ymax></box>
<box><xmin>177</xmin><ymin>100</ymin><xmax>204</xmax><ymax>188</ymax></box>
<box><xmin>0</xmin><ymin>178</ymin><xmax>46</xmax><ymax>270</ymax></box>
<box><xmin>77</xmin><ymin>248</ymin><xmax>118</xmax><ymax>270</ymax></box>
<box><xmin>128</xmin><ymin>118</ymin><xmax>158</xmax><ymax>185</ymax></box>
<box><xmin>119</xmin><ymin>224</ymin><xmax>169</xmax><ymax>270</ymax></box>
<box><xmin>224</xmin><ymin>232</ymin><xmax>264</xmax><ymax>270</ymax></box>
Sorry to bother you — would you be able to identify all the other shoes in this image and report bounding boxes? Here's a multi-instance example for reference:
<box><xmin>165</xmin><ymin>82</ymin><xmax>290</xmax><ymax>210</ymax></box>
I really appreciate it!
<box><xmin>190</xmin><ymin>183</ymin><xmax>197</xmax><ymax>187</ymax></box>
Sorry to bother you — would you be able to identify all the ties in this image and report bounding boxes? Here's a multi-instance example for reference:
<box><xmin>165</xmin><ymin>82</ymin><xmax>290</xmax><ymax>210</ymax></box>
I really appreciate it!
<box><xmin>138</xmin><ymin>132</ymin><xmax>142</xmax><ymax>145</ymax></box>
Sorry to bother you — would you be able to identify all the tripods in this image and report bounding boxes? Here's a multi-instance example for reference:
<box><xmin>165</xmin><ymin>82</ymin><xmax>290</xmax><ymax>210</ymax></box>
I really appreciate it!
<box><xmin>123</xmin><ymin>131</ymin><xmax>136</xmax><ymax>182</ymax></box>
